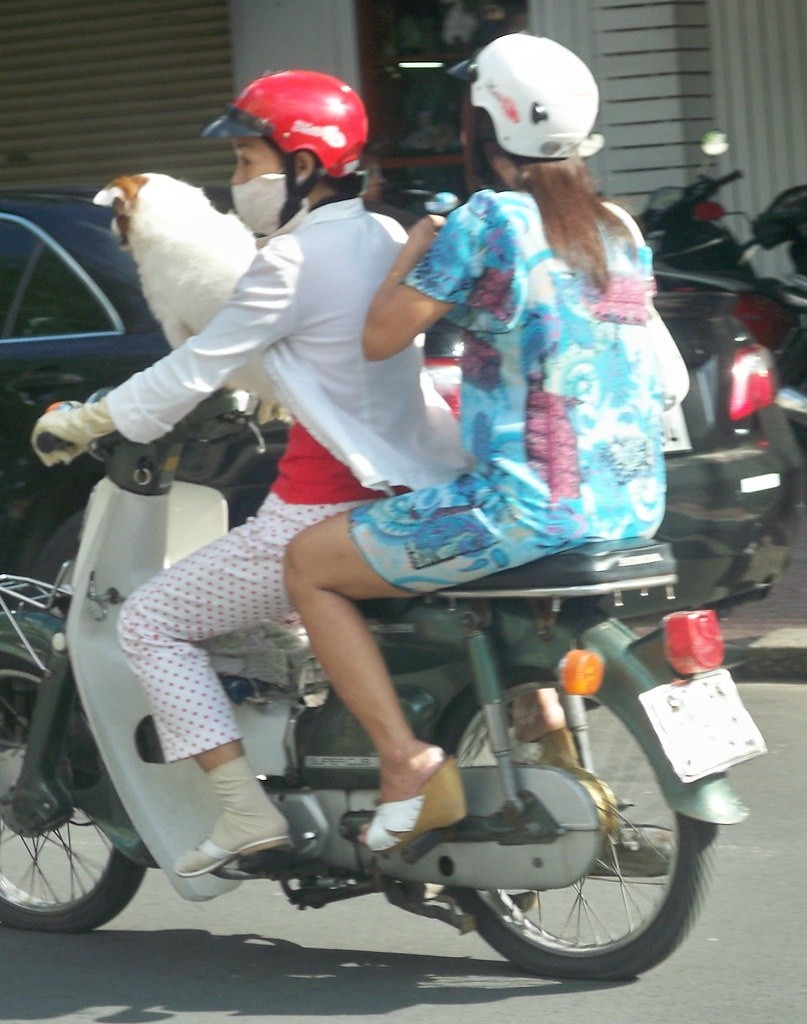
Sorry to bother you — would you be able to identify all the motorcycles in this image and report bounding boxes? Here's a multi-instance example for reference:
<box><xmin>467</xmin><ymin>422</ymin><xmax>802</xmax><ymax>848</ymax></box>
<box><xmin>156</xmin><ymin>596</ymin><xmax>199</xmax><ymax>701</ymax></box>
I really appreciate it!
<box><xmin>0</xmin><ymin>387</ymin><xmax>770</xmax><ymax>983</ymax></box>
<box><xmin>636</xmin><ymin>129</ymin><xmax>807</xmax><ymax>427</ymax></box>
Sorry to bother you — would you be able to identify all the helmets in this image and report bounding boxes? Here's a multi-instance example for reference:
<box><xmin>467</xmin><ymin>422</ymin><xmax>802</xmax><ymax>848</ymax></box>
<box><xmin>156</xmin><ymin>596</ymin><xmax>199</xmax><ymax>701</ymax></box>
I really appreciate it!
<box><xmin>446</xmin><ymin>34</ymin><xmax>604</xmax><ymax>161</ymax></box>
<box><xmin>200</xmin><ymin>72</ymin><xmax>369</xmax><ymax>179</ymax></box>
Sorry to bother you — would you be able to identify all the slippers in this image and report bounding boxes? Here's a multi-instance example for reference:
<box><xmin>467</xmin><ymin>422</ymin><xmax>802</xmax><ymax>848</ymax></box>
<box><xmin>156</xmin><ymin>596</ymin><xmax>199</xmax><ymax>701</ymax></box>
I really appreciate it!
<box><xmin>173</xmin><ymin>835</ymin><xmax>290</xmax><ymax>880</ymax></box>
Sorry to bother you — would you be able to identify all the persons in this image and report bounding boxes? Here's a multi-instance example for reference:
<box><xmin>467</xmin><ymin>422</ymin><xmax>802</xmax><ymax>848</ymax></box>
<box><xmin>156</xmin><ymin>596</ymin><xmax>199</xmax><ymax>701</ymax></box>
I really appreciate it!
<box><xmin>279</xmin><ymin>32</ymin><xmax>689</xmax><ymax>855</ymax></box>
<box><xmin>30</xmin><ymin>70</ymin><xmax>470</xmax><ymax>877</ymax></box>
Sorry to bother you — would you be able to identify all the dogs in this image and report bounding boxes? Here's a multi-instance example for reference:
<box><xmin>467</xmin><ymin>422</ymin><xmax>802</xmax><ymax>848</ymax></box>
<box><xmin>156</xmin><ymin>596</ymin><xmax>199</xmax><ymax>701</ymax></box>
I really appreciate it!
<box><xmin>92</xmin><ymin>172</ymin><xmax>300</xmax><ymax>425</ymax></box>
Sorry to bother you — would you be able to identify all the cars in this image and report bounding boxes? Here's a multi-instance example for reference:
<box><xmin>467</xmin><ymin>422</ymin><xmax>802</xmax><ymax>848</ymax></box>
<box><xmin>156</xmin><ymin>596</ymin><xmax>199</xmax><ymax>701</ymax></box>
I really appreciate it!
<box><xmin>0</xmin><ymin>185</ymin><xmax>805</xmax><ymax>620</ymax></box>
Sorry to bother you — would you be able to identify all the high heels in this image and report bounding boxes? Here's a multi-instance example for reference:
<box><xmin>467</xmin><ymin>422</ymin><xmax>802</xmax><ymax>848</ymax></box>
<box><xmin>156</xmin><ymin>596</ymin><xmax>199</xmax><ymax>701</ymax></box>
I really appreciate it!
<box><xmin>500</xmin><ymin>729</ymin><xmax>579</xmax><ymax>771</ymax></box>
<box><xmin>366</xmin><ymin>756</ymin><xmax>469</xmax><ymax>854</ymax></box>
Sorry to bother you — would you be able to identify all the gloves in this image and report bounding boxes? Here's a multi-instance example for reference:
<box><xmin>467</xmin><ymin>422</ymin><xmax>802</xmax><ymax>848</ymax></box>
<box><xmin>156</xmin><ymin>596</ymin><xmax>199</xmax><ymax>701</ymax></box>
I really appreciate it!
<box><xmin>31</xmin><ymin>398</ymin><xmax>120</xmax><ymax>468</ymax></box>
